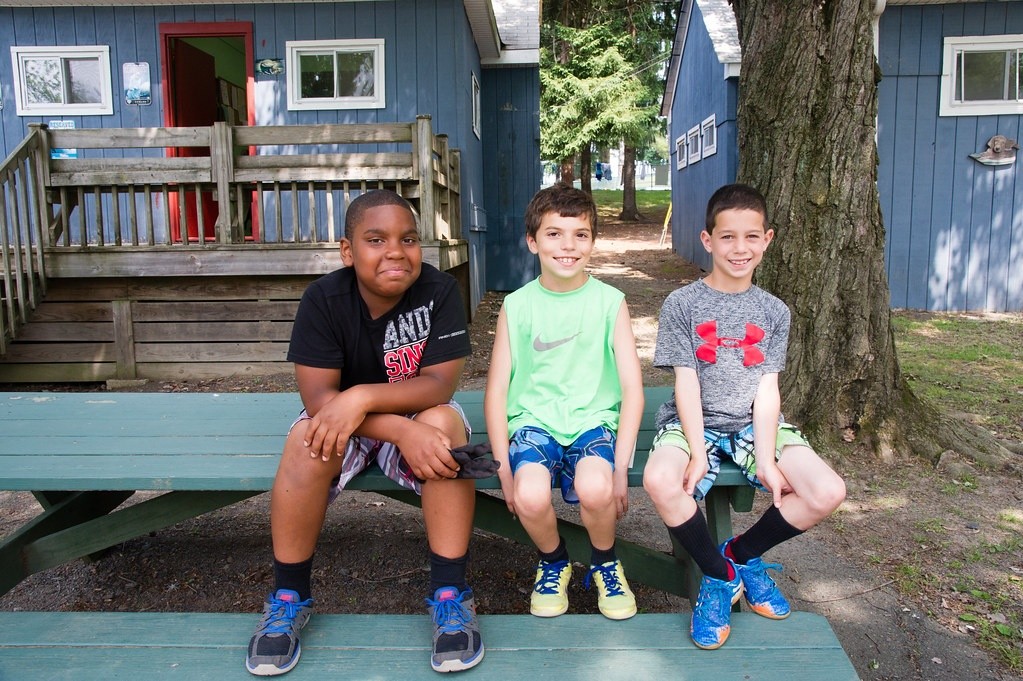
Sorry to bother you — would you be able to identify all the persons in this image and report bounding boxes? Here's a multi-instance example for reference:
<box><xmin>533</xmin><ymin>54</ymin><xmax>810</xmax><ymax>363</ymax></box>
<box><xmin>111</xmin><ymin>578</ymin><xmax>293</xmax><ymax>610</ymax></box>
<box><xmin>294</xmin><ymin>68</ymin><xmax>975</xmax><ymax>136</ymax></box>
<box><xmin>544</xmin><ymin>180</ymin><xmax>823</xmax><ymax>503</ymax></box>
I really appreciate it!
<box><xmin>480</xmin><ymin>183</ymin><xmax>644</xmax><ymax>621</ymax></box>
<box><xmin>631</xmin><ymin>184</ymin><xmax>845</xmax><ymax>649</ymax></box>
<box><xmin>241</xmin><ymin>191</ymin><xmax>488</xmax><ymax>675</ymax></box>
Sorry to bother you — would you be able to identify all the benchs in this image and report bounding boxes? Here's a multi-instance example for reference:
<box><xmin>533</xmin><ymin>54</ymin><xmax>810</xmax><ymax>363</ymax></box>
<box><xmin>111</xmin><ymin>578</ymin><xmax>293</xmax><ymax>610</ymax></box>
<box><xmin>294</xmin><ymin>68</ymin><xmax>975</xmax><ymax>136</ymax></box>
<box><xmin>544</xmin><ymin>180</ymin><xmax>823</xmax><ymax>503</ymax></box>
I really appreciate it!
<box><xmin>0</xmin><ymin>609</ymin><xmax>865</xmax><ymax>681</ymax></box>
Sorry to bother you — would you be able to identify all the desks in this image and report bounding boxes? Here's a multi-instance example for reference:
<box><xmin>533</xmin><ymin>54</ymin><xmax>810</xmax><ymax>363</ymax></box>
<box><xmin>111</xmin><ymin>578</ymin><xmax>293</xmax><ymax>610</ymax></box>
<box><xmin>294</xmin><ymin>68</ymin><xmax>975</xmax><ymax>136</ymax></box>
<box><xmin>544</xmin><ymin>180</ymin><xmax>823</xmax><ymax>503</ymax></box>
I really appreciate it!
<box><xmin>0</xmin><ymin>370</ymin><xmax>777</xmax><ymax>607</ymax></box>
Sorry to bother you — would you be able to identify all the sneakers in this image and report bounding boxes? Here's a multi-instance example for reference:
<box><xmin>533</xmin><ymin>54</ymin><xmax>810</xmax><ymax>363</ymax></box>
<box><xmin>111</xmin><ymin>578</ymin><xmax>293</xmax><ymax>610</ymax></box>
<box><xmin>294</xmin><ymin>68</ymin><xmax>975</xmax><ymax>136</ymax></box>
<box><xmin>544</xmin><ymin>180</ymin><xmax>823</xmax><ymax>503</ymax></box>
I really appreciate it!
<box><xmin>590</xmin><ymin>559</ymin><xmax>637</xmax><ymax>621</ymax></box>
<box><xmin>246</xmin><ymin>585</ymin><xmax>315</xmax><ymax>676</ymax></box>
<box><xmin>529</xmin><ymin>561</ymin><xmax>573</xmax><ymax>617</ymax></box>
<box><xmin>423</xmin><ymin>584</ymin><xmax>485</xmax><ymax>672</ymax></box>
<box><xmin>717</xmin><ymin>534</ymin><xmax>791</xmax><ymax>619</ymax></box>
<box><xmin>689</xmin><ymin>558</ymin><xmax>744</xmax><ymax>650</ymax></box>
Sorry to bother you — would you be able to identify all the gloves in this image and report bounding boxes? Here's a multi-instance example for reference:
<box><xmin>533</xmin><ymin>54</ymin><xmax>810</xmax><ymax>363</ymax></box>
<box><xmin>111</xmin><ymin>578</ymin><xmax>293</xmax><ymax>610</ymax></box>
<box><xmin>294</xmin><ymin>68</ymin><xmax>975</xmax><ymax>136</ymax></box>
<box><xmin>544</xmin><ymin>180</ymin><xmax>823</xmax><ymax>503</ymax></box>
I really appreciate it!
<box><xmin>398</xmin><ymin>442</ymin><xmax>502</xmax><ymax>485</ymax></box>
